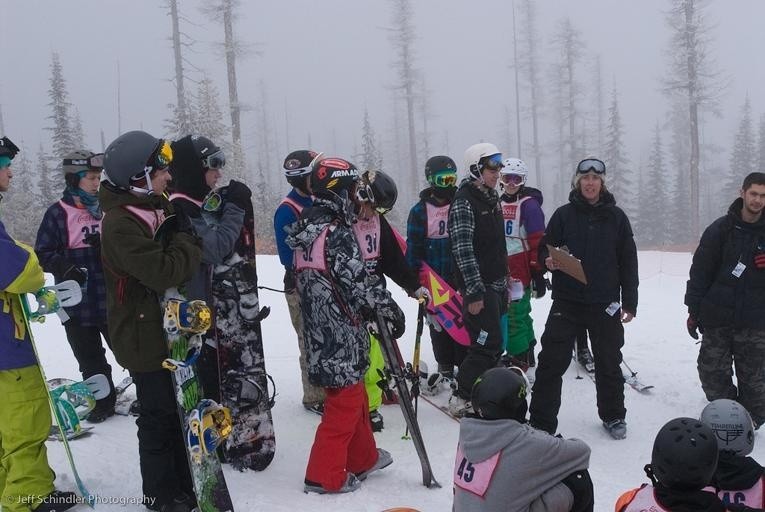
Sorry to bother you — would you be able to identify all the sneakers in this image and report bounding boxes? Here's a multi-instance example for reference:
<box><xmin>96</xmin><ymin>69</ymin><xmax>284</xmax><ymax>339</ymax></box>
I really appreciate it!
<box><xmin>351</xmin><ymin>447</ymin><xmax>392</xmax><ymax>484</ymax></box>
<box><xmin>501</xmin><ymin>340</ymin><xmax>540</xmax><ymax>370</ymax></box>
<box><xmin>86</xmin><ymin>393</ymin><xmax>140</xmax><ymax>425</ymax></box>
<box><xmin>603</xmin><ymin>418</ymin><xmax>629</xmax><ymax>440</ymax></box>
<box><xmin>32</xmin><ymin>489</ymin><xmax>77</xmax><ymax>512</ymax></box>
<box><xmin>302</xmin><ymin>371</ymin><xmax>471</xmax><ymax>434</ymax></box>
<box><xmin>304</xmin><ymin>470</ymin><xmax>360</xmax><ymax>496</ymax></box>
<box><xmin>157</xmin><ymin>491</ymin><xmax>201</xmax><ymax>512</ymax></box>
<box><xmin>575</xmin><ymin>347</ymin><xmax>598</xmax><ymax>373</ymax></box>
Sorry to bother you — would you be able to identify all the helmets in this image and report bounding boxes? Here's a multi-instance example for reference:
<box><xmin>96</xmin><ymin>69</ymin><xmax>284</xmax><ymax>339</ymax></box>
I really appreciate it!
<box><xmin>499</xmin><ymin>157</ymin><xmax>528</xmax><ymax>181</ymax></box>
<box><xmin>470</xmin><ymin>366</ymin><xmax>527</xmax><ymax>422</ymax></box>
<box><xmin>464</xmin><ymin>142</ymin><xmax>502</xmax><ymax>180</ymax></box>
<box><xmin>282</xmin><ymin>149</ymin><xmax>399</xmax><ymax>218</ymax></box>
<box><xmin>425</xmin><ymin>156</ymin><xmax>458</xmax><ymax>186</ymax></box>
<box><xmin>103</xmin><ymin>130</ymin><xmax>173</xmax><ymax>191</ymax></box>
<box><xmin>62</xmin><ymin>149</ymin><xmax>102</xmax><ymax>175</ymax></box>
<box><xmin>699</xmin><ymin>399</ymin><xmax>755</xmax><ymax>459</ymax></box>
<box><xmin>189</xmin><ymin>135</ymin><xmax>222</xmax><ymax>162</ymax></box>
<box><xmin>650</xmin><ymin>416</ymin><xmax>721</xmax><ymax>490</ymax></box>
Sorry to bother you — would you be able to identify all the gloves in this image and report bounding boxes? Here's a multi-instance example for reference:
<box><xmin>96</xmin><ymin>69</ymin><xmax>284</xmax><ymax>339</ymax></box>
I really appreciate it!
<box><xmin>58</xmin><ymin>262</ymin><xmax>88</xmax><ymax>289</ymax></box>
<box><xmin>226</xmin><ymin>178</ymin><xmax>254</xmax><ymax>211</ymax></box>
<box><xmin>166</xmin><ymin>205</ymin><xmax>194</xmax><ymax>234</ymax></box>
<box><xmin>533</xmin><ymin>273</ymin><xmax>547</xmax><ymax>299</ymax></box>
<box><xmin>414</xmin><ymin>285</ymin><xmax>433</xmax><ymax>309</ymax></box>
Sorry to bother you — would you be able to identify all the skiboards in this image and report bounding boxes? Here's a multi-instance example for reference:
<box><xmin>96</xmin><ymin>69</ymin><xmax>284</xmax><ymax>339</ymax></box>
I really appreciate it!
<box><xmin>340</xmin><ymin>187</ymin><xmax>441</xmax><ymax>487</ymax></box>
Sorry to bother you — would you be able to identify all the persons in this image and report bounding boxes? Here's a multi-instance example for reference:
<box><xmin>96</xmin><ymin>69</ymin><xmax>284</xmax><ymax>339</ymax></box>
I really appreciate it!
<box><xmin>2</xmin><ymin>129</ymin><xmax>765</xmax><ymax>510</ymax></box>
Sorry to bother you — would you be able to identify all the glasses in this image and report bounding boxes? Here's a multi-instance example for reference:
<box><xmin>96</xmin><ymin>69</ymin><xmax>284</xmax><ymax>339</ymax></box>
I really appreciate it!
<box><xmin>500</xmin><ymin>173</ymin><xmax>524</xmax><ymax>187</ymax></box>
<box><xmin>506</xmin><ymin>365</ymin><xmax>533</xmax><ymax>396</ymax></box>
<box><xmin>574</xmin><ymin>158</ymin><xmax>606</xmax><ymax>176</ymax></box>
<box><xmin>474</xmin><ymin>152</ymin><xmax>505</xmax><ymax>172</ymax></box>
<box><xmin>201</xmin><ymin>151</ymin><xmax>227</xmax><ymax>171</ymax></box>
<box><xmin>131</xmin><ymin>138</ymin><xmax>172</xmax><ymax>181</ymax></box>
<box><xmin>64</xmin><ymin>153</ymin><xmax>106</xmax><ymax>172</ymax></box>
<box><xmin>427</xmin><ymin>172</ymin><xmax>457</xmax><ymax>189</ymax></box>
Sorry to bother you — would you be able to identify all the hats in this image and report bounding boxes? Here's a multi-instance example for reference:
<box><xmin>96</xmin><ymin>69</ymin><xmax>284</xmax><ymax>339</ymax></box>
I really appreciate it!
<box><xmin>571</xmin><ymin>155</ymin><xmax>606</xmax><ymax>189</ymax></box>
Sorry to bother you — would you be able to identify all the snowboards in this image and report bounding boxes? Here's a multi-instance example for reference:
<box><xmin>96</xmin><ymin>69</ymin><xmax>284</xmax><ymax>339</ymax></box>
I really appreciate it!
<box><xmin>153</xmin><ymin>215</ymin><xmax>235</xmax><ymax>511</ymax></box>
<box><xmin>203</xmin><ymin>185</ymin><xmax>276</xmax><ymax>470</ymax></box>
<box><xmin>421</xmin><ymin>371</ymin><xmax>478</xmax><ymax>421</ymax></box>
<box><xmin>16</xmin><ymin>280</ymin><xmax>110</xmax><ymax>507</ymax></box>
